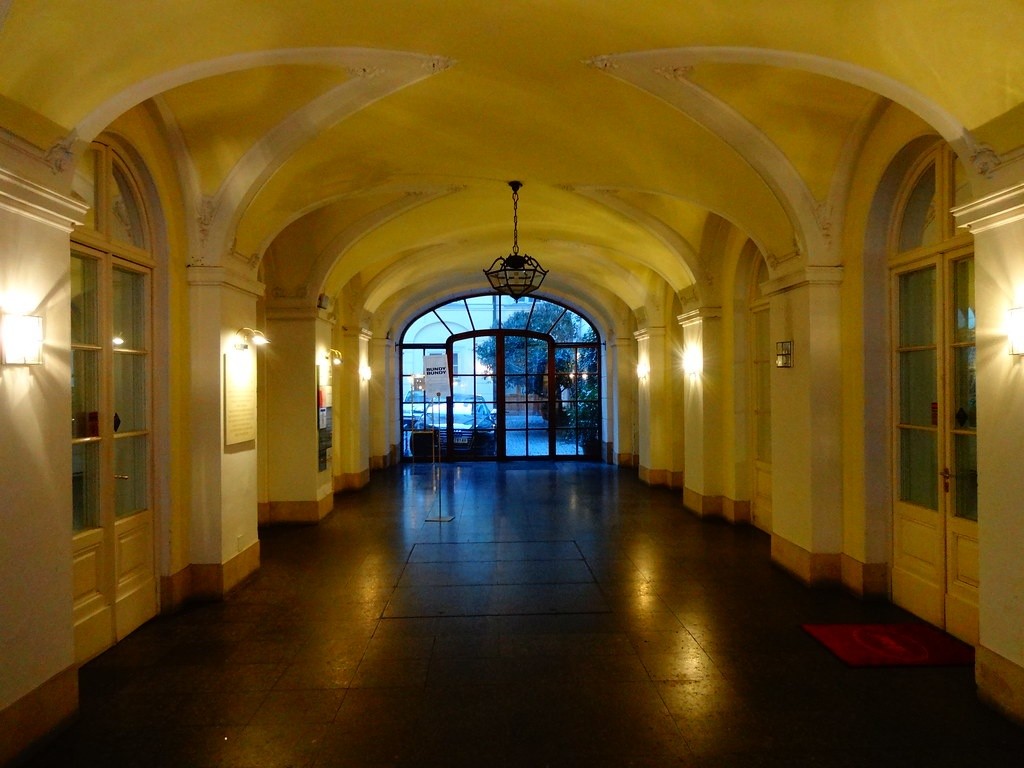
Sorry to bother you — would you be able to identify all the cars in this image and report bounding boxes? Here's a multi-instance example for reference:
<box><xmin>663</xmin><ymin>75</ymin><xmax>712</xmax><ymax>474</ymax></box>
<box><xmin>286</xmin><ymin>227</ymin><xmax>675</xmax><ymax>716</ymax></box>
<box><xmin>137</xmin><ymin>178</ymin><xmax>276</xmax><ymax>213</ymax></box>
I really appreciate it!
<box><xmin>409</xmin><ymin>394</ymin><xmax>497</xmax><ymax>457</ymax></box>
<box><xmin>403</xmin><ymin>390</ymin><xmax>430</xmax><ymax>431</ymax></box>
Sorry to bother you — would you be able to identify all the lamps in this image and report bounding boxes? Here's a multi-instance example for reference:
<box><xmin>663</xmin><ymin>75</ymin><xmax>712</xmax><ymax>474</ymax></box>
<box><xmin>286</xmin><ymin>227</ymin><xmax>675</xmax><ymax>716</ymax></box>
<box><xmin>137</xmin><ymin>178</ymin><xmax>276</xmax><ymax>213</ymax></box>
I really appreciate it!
<box><xmin>232</xmin><ymin>328</ymin><xmax>270</xmax><ymax>354</ymax></box>
<box><xmin>482</xmin><ymin>180</ymin><xmax>549</xmax><ymax>303</ymax></box>
<box><xmin>775</xmin><ymin>341</ymin><xmax>795</xmax><ymax>368</ymax></box>
<box><xmin>326</xmin><ymin>348</ymin><xmax>342</xmax><ymax>365</ymax></box>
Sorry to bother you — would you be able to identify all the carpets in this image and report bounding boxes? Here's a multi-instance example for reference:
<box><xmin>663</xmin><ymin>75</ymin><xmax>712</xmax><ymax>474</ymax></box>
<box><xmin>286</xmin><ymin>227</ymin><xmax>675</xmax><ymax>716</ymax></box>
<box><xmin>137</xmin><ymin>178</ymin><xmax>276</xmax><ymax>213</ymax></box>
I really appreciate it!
<box><xmin>803</xmin><ymin>622</ymin><xmax>974</xmax><ymax>669</ymax></box>
